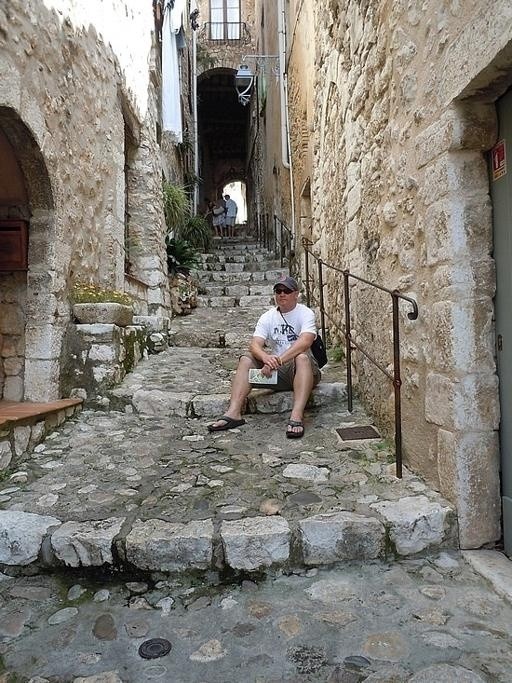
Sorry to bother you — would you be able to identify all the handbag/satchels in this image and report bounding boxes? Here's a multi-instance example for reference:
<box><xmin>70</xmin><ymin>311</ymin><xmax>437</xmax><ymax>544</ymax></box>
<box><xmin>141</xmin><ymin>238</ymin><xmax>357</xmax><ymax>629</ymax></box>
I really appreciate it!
<box><xmin>310</xmin><ymin>333</ymin><xmax>328</xmax><ymax>369</ymax></box>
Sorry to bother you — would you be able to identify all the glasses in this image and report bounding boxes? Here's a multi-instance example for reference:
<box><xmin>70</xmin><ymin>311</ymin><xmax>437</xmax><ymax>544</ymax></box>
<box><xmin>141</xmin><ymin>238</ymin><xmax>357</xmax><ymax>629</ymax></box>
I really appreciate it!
<box><xmin>273</xmin><ymin>287</ymin><xmax>293</xmax><ymax>294</ymax></box>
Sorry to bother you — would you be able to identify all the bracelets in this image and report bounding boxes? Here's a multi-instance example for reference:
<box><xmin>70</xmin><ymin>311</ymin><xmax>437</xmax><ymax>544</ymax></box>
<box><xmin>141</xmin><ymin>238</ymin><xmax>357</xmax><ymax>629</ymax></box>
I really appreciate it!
<box><xmin>277</xmin><ymin>357</ymin><xmax>282</xmax><ymax>365</ymax></box>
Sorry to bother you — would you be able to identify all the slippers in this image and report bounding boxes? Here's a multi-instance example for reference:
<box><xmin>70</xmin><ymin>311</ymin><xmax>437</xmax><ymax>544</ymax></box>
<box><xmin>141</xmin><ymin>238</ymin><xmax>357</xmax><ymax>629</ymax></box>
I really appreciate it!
<box><xmin>285</xmin><ymin>420</ymin><xmax>305</xmax><ymax>438</ymax></box>
<box><xmin>206</xmin><ymin>415</ymin><xmax>246</xmax><ymax>430</ymax></box>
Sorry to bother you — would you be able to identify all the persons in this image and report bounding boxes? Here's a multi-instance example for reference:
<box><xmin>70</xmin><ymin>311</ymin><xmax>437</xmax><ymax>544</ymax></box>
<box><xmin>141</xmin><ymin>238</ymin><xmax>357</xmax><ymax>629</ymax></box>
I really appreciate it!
<box><xmin>225</xmin><ymin>194</ymin><xmax>238</xmax><ymax>236</ymax></box>
<box><xmin>203</xmin><ymin>197</ymin><xmax>223</xmax><ymax>236</ymax></box>
<box><xmin>220</xmin><ymin>196</ymin><xmax>228</xmax><ymax>236</ymax></box>
<box><xmin>206</xmin><ymin>276</ymin><xmax>322</xmax><ymax>438</ymax></box>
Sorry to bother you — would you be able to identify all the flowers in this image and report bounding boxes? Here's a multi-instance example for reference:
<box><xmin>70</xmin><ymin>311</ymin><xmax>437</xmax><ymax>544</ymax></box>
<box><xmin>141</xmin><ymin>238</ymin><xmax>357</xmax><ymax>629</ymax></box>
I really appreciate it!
<box><xmin>68</xmin><ymin>281</ymin><xmax>133</xmax><ymax>306</ymax></box>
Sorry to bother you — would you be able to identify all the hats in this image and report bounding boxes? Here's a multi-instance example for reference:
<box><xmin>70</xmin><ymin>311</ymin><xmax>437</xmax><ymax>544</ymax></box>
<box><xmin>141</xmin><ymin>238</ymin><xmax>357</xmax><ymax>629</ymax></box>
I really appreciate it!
<box><xmin>271</xmin><ymin>276</ymin><xmax>298</xmax><ymax>291</ymax></box>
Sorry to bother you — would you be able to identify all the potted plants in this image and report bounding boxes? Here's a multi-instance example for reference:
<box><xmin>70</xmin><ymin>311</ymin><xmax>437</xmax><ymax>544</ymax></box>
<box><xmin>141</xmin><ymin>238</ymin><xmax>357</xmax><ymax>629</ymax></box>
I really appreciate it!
<box><xmin>167</xmin><ymin>238</ymin><xmax>197</xmax><ymax>276</ymax></box>
